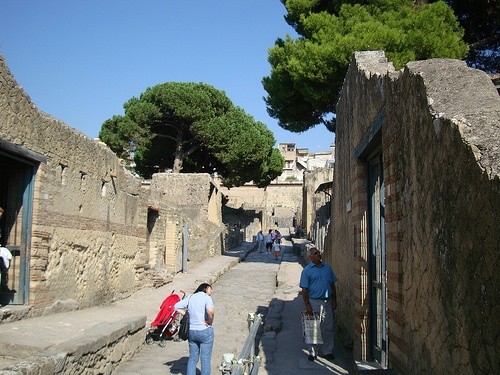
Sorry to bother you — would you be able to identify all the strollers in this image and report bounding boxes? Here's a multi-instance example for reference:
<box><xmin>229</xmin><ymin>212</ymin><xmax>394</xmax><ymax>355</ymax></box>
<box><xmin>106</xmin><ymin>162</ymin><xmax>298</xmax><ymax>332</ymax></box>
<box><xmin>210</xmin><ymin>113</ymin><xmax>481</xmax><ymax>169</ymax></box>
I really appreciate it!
<box><xmin>148</xmin><ymin>290</ymin><xmax>188</xmax><ymax>343</ymax></box>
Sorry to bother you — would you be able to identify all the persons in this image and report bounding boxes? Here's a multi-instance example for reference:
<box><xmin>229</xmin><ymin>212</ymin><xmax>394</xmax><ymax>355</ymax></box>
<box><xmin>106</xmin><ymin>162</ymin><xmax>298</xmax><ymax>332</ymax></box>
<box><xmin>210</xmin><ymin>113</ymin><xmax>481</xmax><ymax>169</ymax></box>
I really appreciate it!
<box><xmin>299</xmin><ymin>246</ymin><xmax>340</xmax><ymax>362</ymax></box>
<box><xmin>173</xmin><ymin>283</ymin><xmax>215</xmax><ymax>374</ymax></box>
<box><xmin>256</xmin><ymin>227</ymin><xmax>283</xmax><ymax>261</ymax></box>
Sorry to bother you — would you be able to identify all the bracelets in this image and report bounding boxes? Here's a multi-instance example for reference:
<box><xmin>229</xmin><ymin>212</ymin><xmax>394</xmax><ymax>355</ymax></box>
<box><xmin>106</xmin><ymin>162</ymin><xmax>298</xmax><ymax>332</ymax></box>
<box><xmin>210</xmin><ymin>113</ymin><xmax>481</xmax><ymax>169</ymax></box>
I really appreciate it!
<box><xmin>304</xmin><ymin>300</ymin><xmax>310</xmax><ymax>305</ymax></box>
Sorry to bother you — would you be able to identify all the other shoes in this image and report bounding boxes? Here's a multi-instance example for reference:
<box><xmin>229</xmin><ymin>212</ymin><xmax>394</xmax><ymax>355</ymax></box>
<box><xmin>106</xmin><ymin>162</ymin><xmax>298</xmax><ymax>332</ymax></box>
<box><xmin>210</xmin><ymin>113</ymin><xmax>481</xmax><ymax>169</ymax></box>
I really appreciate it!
<box><xmin>259</xmin><ymin>251</ymin><xmax>279</xmax><ymax>261</ymax></box>
<box><xmin>308</xmin><ymin>355</ymin><xmax>317</xmax><ymax>361</ymax></box>
<box><xmin>323</xmin><ymin>354</ymin><xmax>334</xmax><ymax>360</ymax></box>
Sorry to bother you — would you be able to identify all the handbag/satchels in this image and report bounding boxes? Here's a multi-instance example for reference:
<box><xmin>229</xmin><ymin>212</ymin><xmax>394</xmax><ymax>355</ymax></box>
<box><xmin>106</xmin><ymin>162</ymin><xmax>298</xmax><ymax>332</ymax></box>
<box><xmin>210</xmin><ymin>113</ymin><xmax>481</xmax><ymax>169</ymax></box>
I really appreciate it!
<box><xmin>304</xmin><ymin>311</ymin><xmax>324</xmax><ymax>345</ymax></box>
<box><xmin>179</xmin><ymin>310</ymin><xmax>190</xmax><ymax>341</ymax></box>
